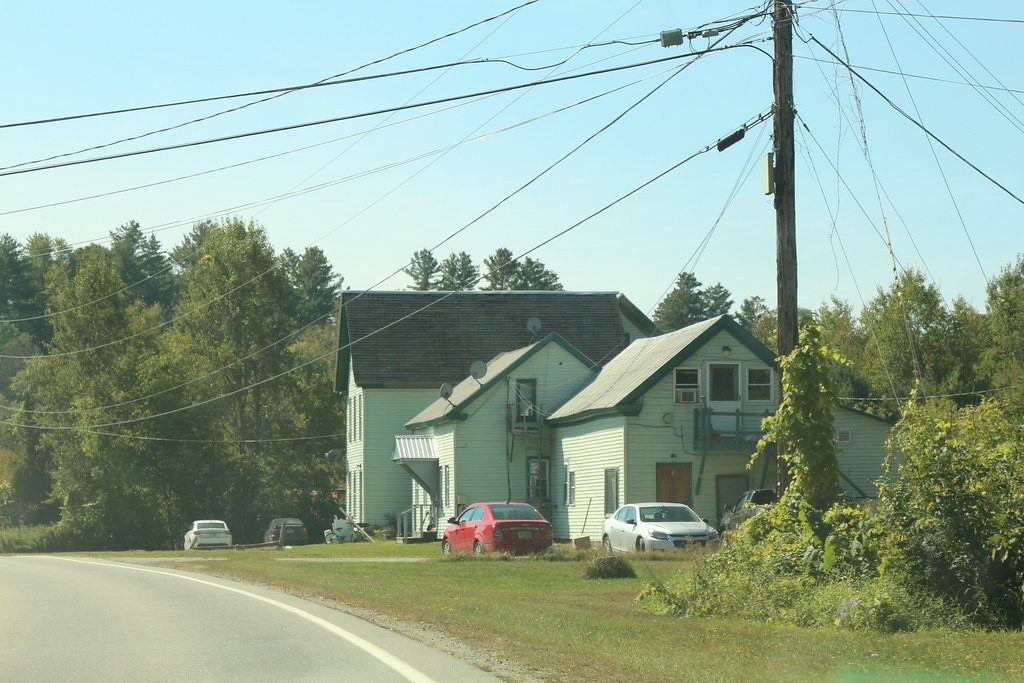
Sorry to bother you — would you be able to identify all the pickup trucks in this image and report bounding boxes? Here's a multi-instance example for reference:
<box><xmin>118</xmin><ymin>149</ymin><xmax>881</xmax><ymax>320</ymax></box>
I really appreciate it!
<box><xmin>719</xmin><ymin>488</ymin><xmax>779</xmax><ymax>553</ymax></box>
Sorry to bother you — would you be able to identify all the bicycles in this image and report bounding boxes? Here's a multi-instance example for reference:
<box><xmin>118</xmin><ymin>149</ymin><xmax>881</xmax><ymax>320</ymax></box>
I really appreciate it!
<box><xmin>324</xmin><ymin>527</ymin><xmax>346</xmax><ymax>544</ymax></box>
<box><xmin>346</xmin><ymin>523</ymin><xmax>363</xmax><ymax>543</ymax></box>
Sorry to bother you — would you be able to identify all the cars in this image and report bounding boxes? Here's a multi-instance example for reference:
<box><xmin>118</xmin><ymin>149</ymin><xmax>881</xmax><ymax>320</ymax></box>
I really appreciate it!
<box><xmin>261</xmin><ymin>518</ymin><xmax>308</xmax><ymax>545</ymax></box>
<box><xmin>180</xmin><ymin>520</ymin><xmax>232</xmax><ymax>550</ymax></box>
<box><xmin>442</xmin><ymin>502</ymin><xmax>553</xmax><ymax>559</ymax></box>
<box><xmin>601</xmin><ymin>502</ymin><xmax>720</xmax><ymax>559</ymax></box>
